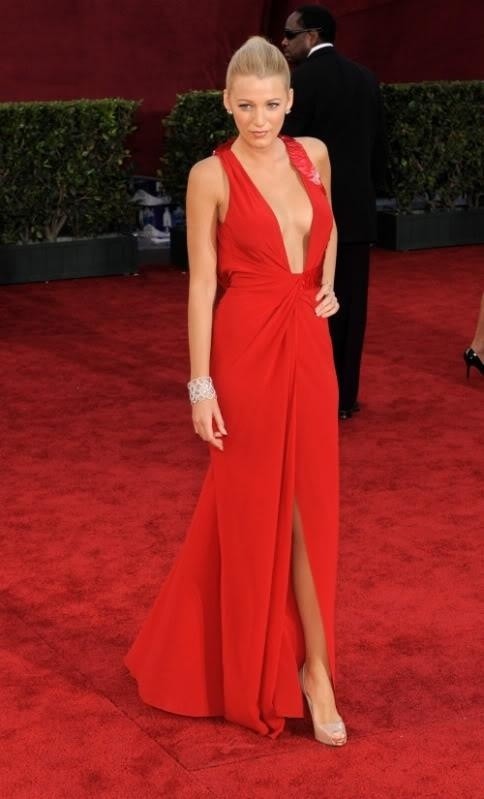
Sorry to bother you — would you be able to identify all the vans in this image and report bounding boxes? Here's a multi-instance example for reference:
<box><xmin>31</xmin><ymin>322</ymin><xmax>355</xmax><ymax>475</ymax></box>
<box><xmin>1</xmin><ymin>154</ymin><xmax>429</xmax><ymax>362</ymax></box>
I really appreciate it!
<box><xmin>297</xmin><ymin>662</ymin><xmax>349</xmax><ymax>747</ymax></box>
<box><xmin>462</xmin><ymin>345</ymin><xmax>484</xmax><ymax>377</ymax></box>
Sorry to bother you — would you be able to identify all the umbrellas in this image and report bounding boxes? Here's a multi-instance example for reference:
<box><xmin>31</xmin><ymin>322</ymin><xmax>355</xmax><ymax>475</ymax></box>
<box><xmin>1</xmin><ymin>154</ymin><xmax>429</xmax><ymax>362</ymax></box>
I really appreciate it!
<box><xmin>340</xmin><ymin>401</ymin><xmax>361</xmax><ymax>419</ymax></box>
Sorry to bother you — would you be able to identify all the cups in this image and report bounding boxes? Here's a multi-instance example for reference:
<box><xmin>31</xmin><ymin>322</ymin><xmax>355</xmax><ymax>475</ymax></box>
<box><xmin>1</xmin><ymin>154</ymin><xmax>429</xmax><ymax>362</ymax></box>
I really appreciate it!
<box><xmin>283</xmin><ymin>27</ymin><xmax>322</xmax><ymax>40</ymax></box>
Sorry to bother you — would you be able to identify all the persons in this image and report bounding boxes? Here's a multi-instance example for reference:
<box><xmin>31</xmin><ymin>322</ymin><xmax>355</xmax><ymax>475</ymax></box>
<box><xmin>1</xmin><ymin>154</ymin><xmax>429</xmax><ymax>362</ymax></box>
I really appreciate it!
<box><xmin>280</xmin><ymin>7</ymin><xmax>384</xmax><ymax>421</ymax></box>
<box><xmin>464</xmin><ymin>293</ymin><xmax>483</xmax><ymax>380</ymax></box>
<box><xmin>124</xmin><ymin>34</ymin><xmax>351</xmax><ymax>748</ymax></box>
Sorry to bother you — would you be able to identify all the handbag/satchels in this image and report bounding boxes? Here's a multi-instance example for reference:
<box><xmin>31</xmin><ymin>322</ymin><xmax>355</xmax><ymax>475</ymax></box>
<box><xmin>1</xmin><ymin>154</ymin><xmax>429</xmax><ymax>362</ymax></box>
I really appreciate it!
<box><xmin>186</xmin><ymin>375</ymin><xmax>219</xmax><ymax>406</ymax></box>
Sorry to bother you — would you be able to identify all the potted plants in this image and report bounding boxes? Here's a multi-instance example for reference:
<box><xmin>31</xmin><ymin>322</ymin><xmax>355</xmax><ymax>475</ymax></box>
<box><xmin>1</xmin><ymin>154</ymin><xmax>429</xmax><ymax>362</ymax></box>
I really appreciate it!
<box><xmin>376</xmin><ymin>85</ymin><xmax>482</xmax><ymax>252</ymax></box>
<box><xmin>2</xmin><ymin>98</ymin><xmax>138</xmax><ymax>283</ymax></box>
<box><xmin>161</xmin><ymin>91</ymin><xmax>237</xmax><ymax>266</ymax></box>
